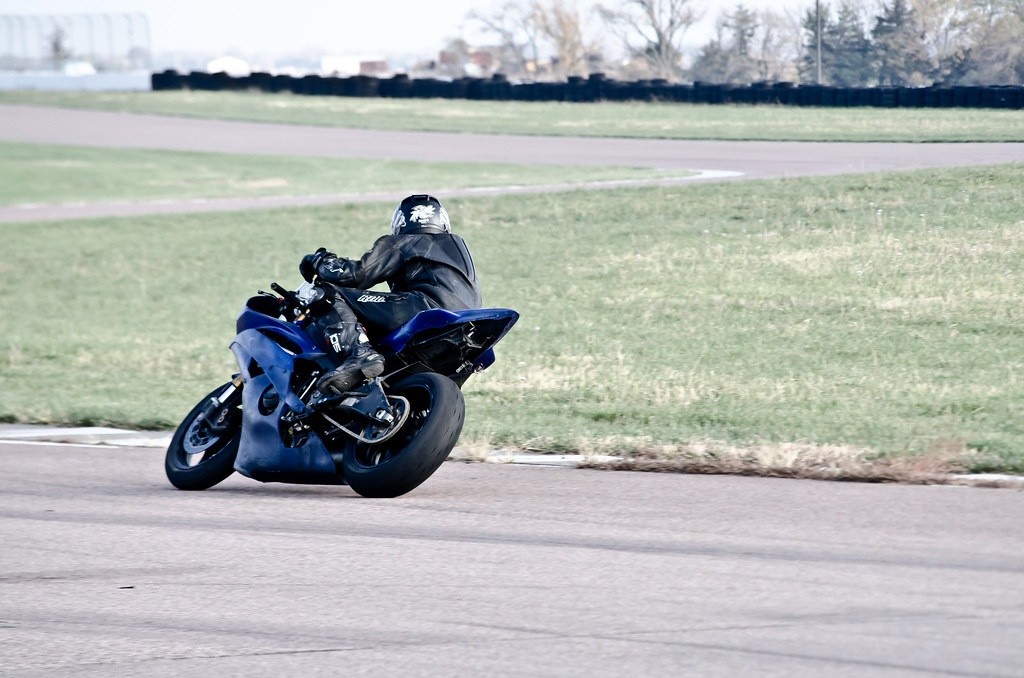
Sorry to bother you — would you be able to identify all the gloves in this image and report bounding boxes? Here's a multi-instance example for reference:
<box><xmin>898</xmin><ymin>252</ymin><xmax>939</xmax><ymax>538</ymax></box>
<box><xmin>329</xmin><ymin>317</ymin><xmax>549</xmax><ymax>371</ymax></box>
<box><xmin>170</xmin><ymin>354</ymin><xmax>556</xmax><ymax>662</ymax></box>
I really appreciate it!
<box><xmin>299</xmin><ymin>247</ymin><xmax>337</xmax><ymax>284</ymax></box>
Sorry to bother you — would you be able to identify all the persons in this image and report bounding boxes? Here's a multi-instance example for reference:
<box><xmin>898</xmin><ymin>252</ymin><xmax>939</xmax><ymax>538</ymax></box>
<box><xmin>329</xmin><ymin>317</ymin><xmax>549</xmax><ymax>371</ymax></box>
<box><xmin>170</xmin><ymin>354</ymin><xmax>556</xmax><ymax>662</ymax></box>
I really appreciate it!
<box><xmin>299</xmin><ymin>194</ymin><xmax>483</xmax><ymax>395</ymax></box>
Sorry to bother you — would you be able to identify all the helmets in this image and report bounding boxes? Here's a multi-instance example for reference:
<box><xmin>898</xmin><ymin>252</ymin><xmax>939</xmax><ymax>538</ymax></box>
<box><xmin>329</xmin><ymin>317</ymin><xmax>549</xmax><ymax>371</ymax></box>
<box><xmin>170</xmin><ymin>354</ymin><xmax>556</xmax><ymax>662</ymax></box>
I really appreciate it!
<box><xmin>391</xmin><ymin>194</ymin><xmax>451</xmax><ymax>236</ymax></box>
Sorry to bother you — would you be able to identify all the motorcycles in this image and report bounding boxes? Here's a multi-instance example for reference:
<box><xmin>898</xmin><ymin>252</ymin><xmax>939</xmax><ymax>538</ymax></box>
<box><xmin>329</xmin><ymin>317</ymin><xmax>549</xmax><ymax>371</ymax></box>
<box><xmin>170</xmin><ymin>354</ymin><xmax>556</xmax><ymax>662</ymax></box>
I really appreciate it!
<box><xmin>164</xmin><ymin>248</ymin><xmax>521</xmax><ymax>498</ymax></box>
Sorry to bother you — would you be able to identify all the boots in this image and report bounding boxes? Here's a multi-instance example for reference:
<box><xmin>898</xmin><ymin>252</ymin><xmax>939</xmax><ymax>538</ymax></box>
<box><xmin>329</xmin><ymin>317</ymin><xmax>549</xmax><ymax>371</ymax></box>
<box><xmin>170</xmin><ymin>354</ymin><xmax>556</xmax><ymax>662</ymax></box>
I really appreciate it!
<box><xmin>316</xmin><ymin>301</ymin><xmax>385</xmax><ymax>394</ymax></box>
